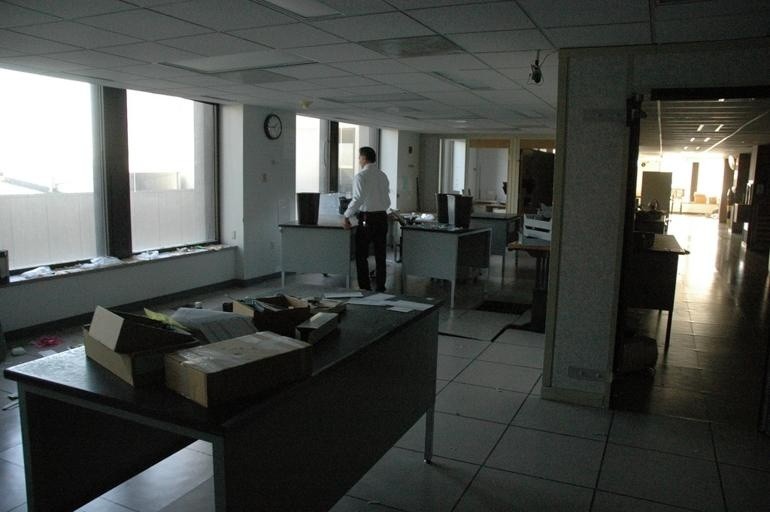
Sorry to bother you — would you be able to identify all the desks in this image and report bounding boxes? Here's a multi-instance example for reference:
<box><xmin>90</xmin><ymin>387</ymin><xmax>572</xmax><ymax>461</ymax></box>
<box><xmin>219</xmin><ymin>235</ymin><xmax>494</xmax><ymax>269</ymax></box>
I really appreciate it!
<box><xmin>621</xmin><ymin>234</ymin><xmax>685</xmax><ymax>353</ymax></box>
<box><xmin>636</xmin><ymin>213</ymin><xmax>666</xmax><ymax>235</ymax></box>
<box><xmin>3</xmin><ymin>282</ymin><xmax>445</xmax><ymax>512</ymax></box>
<box><xmin>279</xmin><ymin>211</ymin><xmax>551</xmax><ymax>309</ymax></box>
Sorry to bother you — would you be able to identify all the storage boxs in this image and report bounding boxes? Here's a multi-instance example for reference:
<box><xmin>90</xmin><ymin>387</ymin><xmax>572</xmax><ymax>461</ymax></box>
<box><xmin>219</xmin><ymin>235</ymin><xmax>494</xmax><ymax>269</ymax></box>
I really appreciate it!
<box><xmin>83</xmin><ymin>293</ymin><xmax>339</xmax><ymax>408</ymax></box>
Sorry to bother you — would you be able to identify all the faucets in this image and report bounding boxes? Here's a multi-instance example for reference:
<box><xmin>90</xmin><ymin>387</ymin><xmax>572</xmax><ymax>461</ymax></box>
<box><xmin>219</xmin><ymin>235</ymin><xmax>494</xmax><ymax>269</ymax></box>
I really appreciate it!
<box><xmin>648</xmin><ymin>199</ymin><xmax>661</xmax><ymax>209</ymax></box>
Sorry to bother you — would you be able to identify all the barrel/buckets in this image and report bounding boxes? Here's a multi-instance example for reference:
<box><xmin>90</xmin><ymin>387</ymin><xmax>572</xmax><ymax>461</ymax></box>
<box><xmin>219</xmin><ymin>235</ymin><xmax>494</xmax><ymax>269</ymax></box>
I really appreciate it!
<box><xmin>295</xmin><ymin>192</ymin><xmax>320</xmax><ymax>226</ymax></box>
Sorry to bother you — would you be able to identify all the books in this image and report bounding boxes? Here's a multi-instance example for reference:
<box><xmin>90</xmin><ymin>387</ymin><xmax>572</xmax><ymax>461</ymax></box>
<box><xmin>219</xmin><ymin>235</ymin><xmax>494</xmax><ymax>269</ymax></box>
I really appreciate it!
<box><xmin>253</xmin><ymin>299</ymin><xmax>289</xmax><ymax>314</ymax></box>
<box><xmin>168</xmin><ymin>306</ymin><xmax>260</xmax><ymax>346</ymax></box>
<box><xmin>302</xmin><ymin>296</ymin><xmax>349</xmax><ymax>315</ymax></box>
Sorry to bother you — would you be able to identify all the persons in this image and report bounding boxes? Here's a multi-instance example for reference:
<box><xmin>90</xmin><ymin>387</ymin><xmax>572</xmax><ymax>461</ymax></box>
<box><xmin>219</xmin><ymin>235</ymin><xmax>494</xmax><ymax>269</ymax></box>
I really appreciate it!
<box><xmin>341</xmin><ymin>146</ymin><xmax>391</xmax><ymax>294</ymax></box>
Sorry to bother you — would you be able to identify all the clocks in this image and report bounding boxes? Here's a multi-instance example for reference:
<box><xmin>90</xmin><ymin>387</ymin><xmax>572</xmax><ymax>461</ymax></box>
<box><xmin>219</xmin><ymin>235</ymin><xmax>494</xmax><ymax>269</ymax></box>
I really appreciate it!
<box><xmin>265</xmin><ymin>114</ymin><xmax>282</xmax><ymax>140</ymax></box>
